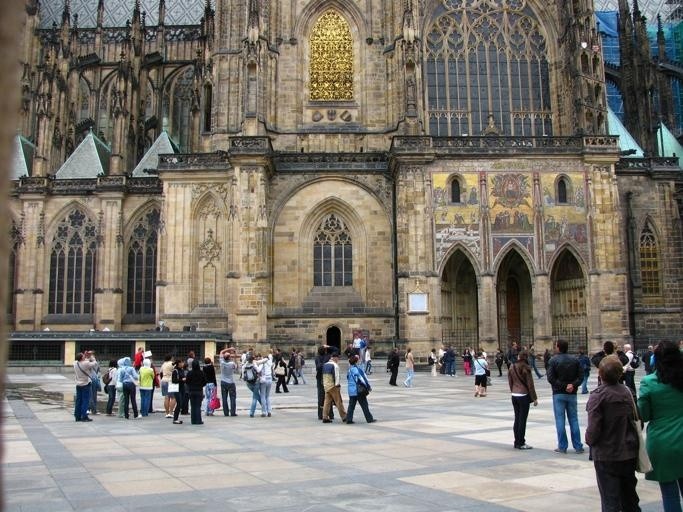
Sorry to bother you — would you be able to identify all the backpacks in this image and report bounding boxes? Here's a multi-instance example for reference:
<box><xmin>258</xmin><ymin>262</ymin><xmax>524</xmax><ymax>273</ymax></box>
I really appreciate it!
<box><xmin>630</xmin><ymin>352</ymin><xmax>640</xmax><ymax>368</ymax></box>
<box><xmin>102</xmin><ymin>367</ymin><xmax>113</xmax><ymax>385</ymax></box>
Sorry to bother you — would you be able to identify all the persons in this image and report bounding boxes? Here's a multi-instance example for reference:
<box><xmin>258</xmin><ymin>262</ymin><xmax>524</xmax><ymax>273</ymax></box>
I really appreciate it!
<box><xmin>70</xmin><ymin>333</ymin><xmax>377</xmax><ymax>424</ymax></box>
<box><xmin>585</xmin><ymin>354</ymin><xmax>641</xmax><ymax>512</ymax></box>
<box><xmin>545</xmin><ymin>339</ymin><xmax>585</xmax><ymax>454</ymax></box>
<box><xmin>403</xmin><ymin>348</ymin><xmax>414</xmax><ymax>387</ymax></box>
<box><xmin>385</xmin><ymin>346</ymin><xmax>399</xmax><ymax>387</ymax></box>
<box><xmin>426</xmin><ymin>339</ymin><xmax>683</xmax><ymax>392</ymax></box>
<box><xmin>473</xmin><ymin>351</ymin><xmax>487</xmax><ymax>397</ymax></box>
<box><xmin>507</xmin><ymin>348</ymin><xmax>539</xmax><ymax>449</ymax></box>
<box><xmin>635</xmin><ymin>339</ymin><xmax>683</xmax><ymax>512</ymax></box>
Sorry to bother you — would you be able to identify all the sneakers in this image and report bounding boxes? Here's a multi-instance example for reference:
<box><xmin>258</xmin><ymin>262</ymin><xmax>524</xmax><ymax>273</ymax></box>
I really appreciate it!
<box><xmin>165</xmin><ymin>413</ymin><xmax>215</xmax><ymax>424</ymax></box>
<box><xmin>515</xmin><ymin>444</ymin><xmax>532</xmax><ymax>449</ymax></box>
<box><xmin>554</xmin><ymin>448</ymin><xmax>587</xmax><ymax>454</ymax></box>
<box><xmin>225</xmin><ymin>414</ymin><xmax>237</xmax><ymax>416</ymax></box>
<box><xmin>86</xmin><ymin>408</ymin><xmax>155</xmax><ymax>420</ymax></box>
<box><xmin>318</xmin><ymin>416</ymin><xmax>376</xmax><ymax>424</ymax></box>
<box><xmin>76</xmin><ymin>416</ymin><xmax>92</xmax><ymax>422</ymax></box>
<box><xmin>250</xmin><ymin>413</ymin><xmax>271</xmax><ymax>417</ymax></box>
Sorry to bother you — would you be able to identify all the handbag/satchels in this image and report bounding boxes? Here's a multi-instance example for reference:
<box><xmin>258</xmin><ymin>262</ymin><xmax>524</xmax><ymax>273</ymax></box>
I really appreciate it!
<box><xmin>243</xmin><ymin>366</ymin><xmax>257</xmax><ymax>382</ymax></box>
<box><xmin>275</xmin><ymin>366</ymin><xmax>285</xmax><ymax>375</ymax></box>
<box><xmin>208</xmin><ymin>397</ymin><xmax>220</xmax><ymax>410</ymax></box>
<box><xmin>131</xmin><ymin>374</ymin><xmax>139</xmax><ymax>386</ymax></box>
<box><xmin>357</xmin><ymin>383</ymin><xmax>369</xmax><ymax>396</ymax></box>
<box><xmin>485</xmin><ymin>368</ymin><xmax>490</xmax><ymax>376</ymax></box>
<box><xmin>631</xmin><ymin>420</ymin><xmax>654</xmax><ymax>473</ymax></box>
<box><xmin>168</xmin><ymin>380</ymin><xmax>179</xmax><ymax>392</ymax></box>
<box><xmin>428</xmin><ymin>356</ymin><xmax>434</xmax><ymax>366</ymax></box>
